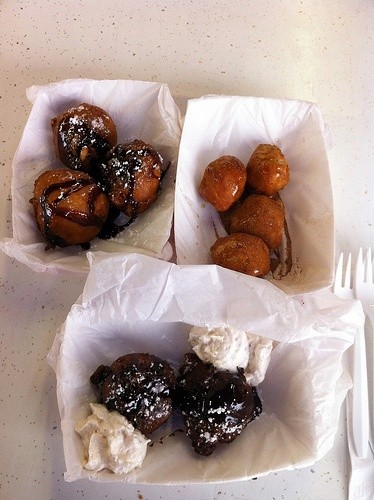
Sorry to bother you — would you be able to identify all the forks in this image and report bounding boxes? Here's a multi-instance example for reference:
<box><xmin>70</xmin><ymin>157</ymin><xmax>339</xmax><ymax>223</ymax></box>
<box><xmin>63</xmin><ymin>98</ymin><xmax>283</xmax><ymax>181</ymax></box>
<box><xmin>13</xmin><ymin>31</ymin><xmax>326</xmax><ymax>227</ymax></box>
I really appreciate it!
<box><xmin>335</xmin><ymin>247</ymin><xmax>373</xmax><ymax>457</ymax></box>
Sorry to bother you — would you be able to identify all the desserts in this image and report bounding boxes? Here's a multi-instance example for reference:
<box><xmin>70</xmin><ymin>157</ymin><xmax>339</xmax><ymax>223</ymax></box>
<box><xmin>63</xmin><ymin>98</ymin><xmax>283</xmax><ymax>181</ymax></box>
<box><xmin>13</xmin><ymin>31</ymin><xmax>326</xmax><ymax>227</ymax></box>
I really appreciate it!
<box><xmin>197</xmin><ymin>143</ymin><xmax>294</xmax><ymax>277</ymax></box>
<box><xmin>72</xmin><ymin>323</ymin><xmax>275</xmax><ymax>480</ymax></box>
<box><xmin>28</xmin><ymin>100</ymin><xmax>165</xmax><ymax>254</ymax></box>
